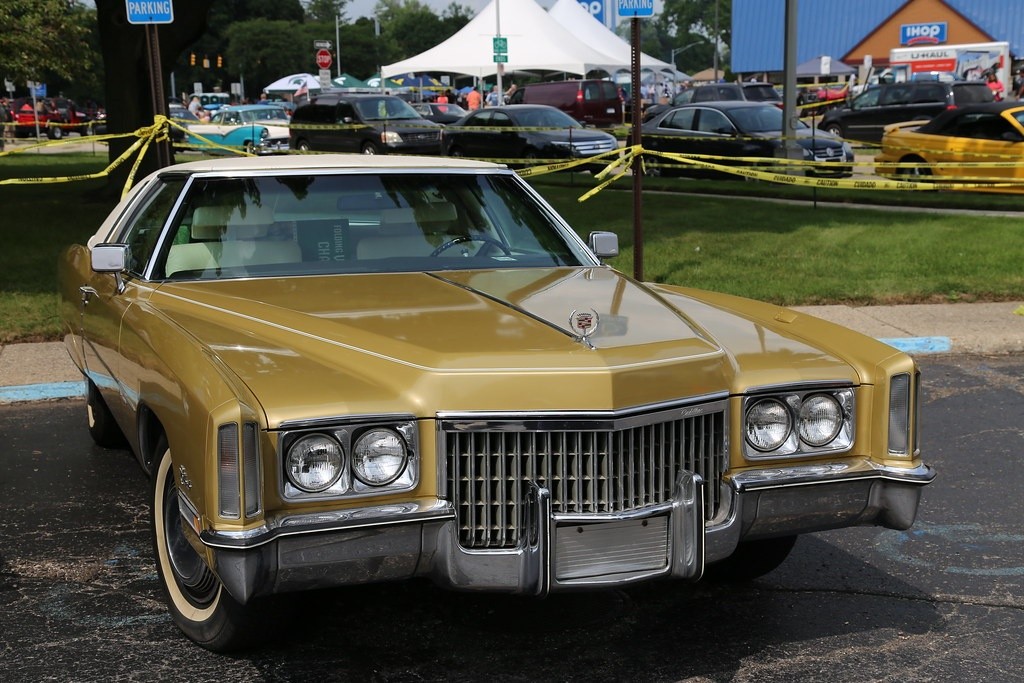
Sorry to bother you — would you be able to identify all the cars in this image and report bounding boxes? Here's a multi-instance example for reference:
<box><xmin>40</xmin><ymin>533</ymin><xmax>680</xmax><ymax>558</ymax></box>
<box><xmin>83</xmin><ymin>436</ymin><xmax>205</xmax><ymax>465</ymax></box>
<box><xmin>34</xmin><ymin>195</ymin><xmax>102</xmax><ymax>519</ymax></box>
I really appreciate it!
<box><xmin>52</xmin><ymin>154</ymin><xmax>936</xmax><ymax>654</ymax></box>
<box><xmin>439</xmin><ymin>103</ymin><xmax>622</xmax><ymax>161</ymax></box>
<box><xmin>289</xmin><ymin>92</ymin><xmax>441</xmax><ymax>155</ymax></box>
<box><xmin>625</xmin><ymin>100</ymin><xmax>854</xmax><ymax>181</ymax></box>
<box><xmin>93</xmin><ymin>103</ymin><xmax>107</xmax><ymax>138</ymax></box>
<box><xmin>167</xmin><ymin>92</ymin><xmax>290</xmax><ymax>153</ymax></box>
<box><xmin>816</xmin><ymin>78</ymin><xmax>994</xmax><ymax>145</ymax></box>
<box><xmin>874</xmin><ymin>101</ymin><xmax>1024</xmax><ymax>197</ymax></box>
<box><xmin>405</xmin><ymin>99</ymin><xmax>472</xmax><ymax>125</ymax></box>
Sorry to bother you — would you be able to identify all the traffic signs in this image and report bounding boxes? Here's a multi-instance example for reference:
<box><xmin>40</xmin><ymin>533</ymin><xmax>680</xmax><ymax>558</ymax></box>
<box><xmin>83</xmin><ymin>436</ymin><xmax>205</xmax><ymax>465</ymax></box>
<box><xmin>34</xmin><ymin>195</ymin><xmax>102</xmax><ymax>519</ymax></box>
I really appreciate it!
<box><xmin>313</xmin><ymin>40</ymin><xmax>334</xmax><ymax>49</ymax></box>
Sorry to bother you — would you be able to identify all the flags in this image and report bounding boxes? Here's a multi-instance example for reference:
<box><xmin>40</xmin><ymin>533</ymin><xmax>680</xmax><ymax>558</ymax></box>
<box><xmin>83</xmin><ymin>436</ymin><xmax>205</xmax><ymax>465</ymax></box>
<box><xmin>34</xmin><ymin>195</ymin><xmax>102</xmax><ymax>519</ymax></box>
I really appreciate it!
<box><xmin>294</xmin><ymin>81</ymin><xmax>308</xmax><ymax>97</ymax></box>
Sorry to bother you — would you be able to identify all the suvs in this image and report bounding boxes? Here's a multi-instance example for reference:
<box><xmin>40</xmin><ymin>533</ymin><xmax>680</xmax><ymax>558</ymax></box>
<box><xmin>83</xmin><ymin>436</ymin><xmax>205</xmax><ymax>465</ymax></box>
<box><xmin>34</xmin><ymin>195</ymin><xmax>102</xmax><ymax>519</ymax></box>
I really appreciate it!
<box><xmin>10</xmin><ymin>96</ymin><xmax>91</xmax><ymax>140</ymax></box>
<box><xmin>638</xmin><ymin>82</ymin><xmax>784</xmax><ymax>127</ymax></box>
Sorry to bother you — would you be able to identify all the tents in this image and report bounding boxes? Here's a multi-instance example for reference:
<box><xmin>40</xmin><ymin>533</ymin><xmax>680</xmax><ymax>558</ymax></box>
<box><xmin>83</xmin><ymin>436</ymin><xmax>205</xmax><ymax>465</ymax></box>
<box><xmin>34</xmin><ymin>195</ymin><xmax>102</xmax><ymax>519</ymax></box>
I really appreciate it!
<box><xmin>381</xmin><ymin>0</ymin><xmax>677</xmax><ymax>100</ymax></box>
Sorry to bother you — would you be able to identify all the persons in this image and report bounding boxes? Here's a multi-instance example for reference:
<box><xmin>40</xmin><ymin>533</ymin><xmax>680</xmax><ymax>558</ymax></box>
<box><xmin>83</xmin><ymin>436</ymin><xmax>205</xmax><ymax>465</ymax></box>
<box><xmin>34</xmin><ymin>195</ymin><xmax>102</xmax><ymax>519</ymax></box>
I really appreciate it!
<box><xmin>257</xmin><ymin>94</ymin><xmax>268</xmax><ymax>104</ymax></box>
<box><xmin>640</xmin><ymin>83</ymin><xmax>671</xmax><ymax>99</ymax></box>
<box><xmin>188</xmin><ymin>96</ymin><xmax>202</xmax><ymax>119</ymax></box>
<box><xmin>719</xmin><ymin>76</ymin><xmax>727</xmax><ymax>83</ymax></box>
<box><xmin>1012</xmin><ymin>64</ymin><xmax>1024</xmax><ymax>100</ymax></box>
<box><xmin>457</xmin><ymin>83</ymin><xmax>517</xmax><ymax>111</ymax></box>
<box><xmin>796</xmin><ymin>88</ymin><xmax>808</xmax><ymax>117</ymax></box>
<box><xmin>0</xmin><ymin>97</ymin><xmax>15</xmax><ymax>143</ymax></box>
<box><xmin>985</xmin><ymin>73</ymin><xmax>1004</xmax><ymax>102</ymax></box>
<box><xmin>56</xmin><ymin>93</ymin><xmax>72</xmax><ymax>123</ymax></box>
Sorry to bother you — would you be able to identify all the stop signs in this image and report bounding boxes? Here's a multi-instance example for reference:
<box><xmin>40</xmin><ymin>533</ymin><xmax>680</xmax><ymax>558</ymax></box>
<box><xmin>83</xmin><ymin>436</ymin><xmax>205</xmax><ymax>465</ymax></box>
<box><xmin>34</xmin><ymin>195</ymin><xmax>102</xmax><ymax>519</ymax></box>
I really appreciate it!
<box><xmin>315</xmin><ymin>48</ymin><xmax>331</xmax><ymax>69</ymax></box>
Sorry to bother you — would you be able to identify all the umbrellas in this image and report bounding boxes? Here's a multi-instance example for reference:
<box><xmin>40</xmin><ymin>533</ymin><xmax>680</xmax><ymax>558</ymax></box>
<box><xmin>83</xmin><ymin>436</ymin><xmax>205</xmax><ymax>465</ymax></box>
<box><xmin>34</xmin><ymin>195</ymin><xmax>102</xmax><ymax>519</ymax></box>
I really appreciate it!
<box><xmin>263</xmin><ymin>72</ymin><xmax>453</xmax><ymax>103</ymax></box>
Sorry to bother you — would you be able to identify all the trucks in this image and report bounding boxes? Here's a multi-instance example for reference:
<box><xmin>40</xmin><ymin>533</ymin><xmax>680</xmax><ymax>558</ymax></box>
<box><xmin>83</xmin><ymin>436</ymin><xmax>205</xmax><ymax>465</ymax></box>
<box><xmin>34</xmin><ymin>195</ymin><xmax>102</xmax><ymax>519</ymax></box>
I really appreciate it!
<box><xmin>849</xmin><ymin>42</ymin><xmax>1013</xmax><ymax>103</ymax></box>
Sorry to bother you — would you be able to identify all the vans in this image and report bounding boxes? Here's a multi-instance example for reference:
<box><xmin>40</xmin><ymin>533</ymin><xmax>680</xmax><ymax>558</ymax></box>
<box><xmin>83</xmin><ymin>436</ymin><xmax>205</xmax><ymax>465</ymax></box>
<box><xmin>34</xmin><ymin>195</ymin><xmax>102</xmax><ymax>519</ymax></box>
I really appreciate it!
<box><xmin>506</xmin><ymin>79</ymin><xmax>624</xmax><ymax>129</ymax></box>
<box><xmin>771</xmin><ymin>72</ymin><xmax>858</xmax><ymax>111</ymax></box>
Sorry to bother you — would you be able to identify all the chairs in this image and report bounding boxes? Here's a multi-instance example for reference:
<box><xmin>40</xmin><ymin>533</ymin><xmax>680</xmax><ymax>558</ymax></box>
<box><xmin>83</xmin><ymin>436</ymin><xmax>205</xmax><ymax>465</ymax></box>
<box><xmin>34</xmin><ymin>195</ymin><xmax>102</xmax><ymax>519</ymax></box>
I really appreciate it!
<box><xmin>165</xmin><ymin>207</ymin><xmax>302</xmax><ymax>279</ymax></box>
<box><xmin>892</xmin><ymin>91</ymin><xmax>904</xmax><ymax>101</ymax></box>
<box><xmin>352</xmin><ymin>203</ymin><xmax>485</xmax><ymax>262</ymax></box>
